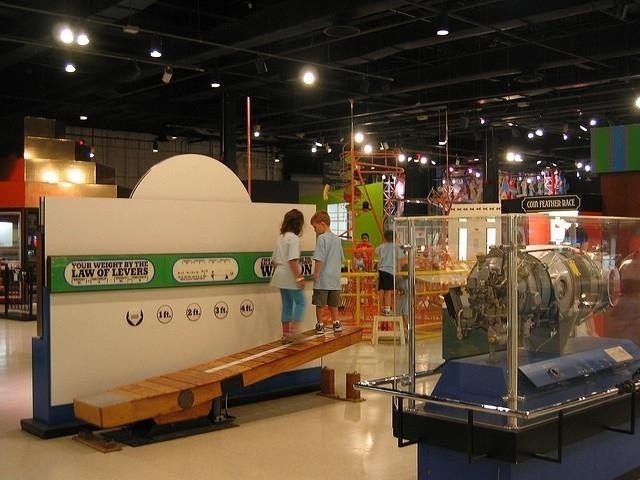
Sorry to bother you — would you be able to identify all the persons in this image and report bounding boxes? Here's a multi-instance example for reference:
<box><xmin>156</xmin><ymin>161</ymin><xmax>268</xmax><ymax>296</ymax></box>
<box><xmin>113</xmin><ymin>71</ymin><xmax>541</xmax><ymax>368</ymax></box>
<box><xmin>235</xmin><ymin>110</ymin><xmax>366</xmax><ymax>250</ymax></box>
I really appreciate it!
<box><xmin>310</xmin><ymin>211</ymin><xmax>344</xmax><ymax>334</ymax></box>
<box><xmin>392</xmin><ymin>172</ymin><xmax>406</xmax><ymax>217</ymax></box>
<box><xmin>269</xmin><ymin>208</ymin><xmax>308</xmax><ymax>345</ymax></box>
<box><xmin>356</xmin><ymin>232</ymin><xmax>375</xmax><ymax>307</ymax></box>
<box><xmin>375</xmin><ymin>230</ymin><xmax>406</xmax><ymax>316</ymax></box>
<box><xmin>397</xmin><ymin>261</ymin><xmax>411</xmax><ymax>339</ymax></box>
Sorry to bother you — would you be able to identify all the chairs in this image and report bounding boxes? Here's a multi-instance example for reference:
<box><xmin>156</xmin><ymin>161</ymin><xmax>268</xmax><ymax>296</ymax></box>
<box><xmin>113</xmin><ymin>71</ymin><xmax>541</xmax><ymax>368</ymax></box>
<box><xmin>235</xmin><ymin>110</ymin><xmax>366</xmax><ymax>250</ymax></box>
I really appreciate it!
<box><xmin>333</xmin><ymin>321</ymin><xmax>344</xmax><ymax>331</ymax></box>
<box><xmin>382</xmin><ymin>309</ymin><xmax>392</xmax><ymax>316</ymax></box>
<box><xmin>316</xmin><ymin>323</ymin><xmax>324</xmax><ymax>334</ymax></box>
<box><xmin>281</xmin><ymin>335</ymin><xmax>293</xmax><ymax>345</ymax></box>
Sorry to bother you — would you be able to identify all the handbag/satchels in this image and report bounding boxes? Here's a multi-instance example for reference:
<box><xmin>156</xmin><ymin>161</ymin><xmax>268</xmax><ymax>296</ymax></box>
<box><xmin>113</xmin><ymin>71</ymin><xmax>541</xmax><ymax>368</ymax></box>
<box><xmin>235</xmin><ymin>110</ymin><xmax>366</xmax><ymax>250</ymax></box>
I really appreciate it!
<box><xmin>296</xmin><ymin>276</ymin><xmax>304</xmax><ymax>283</ymax></box>
